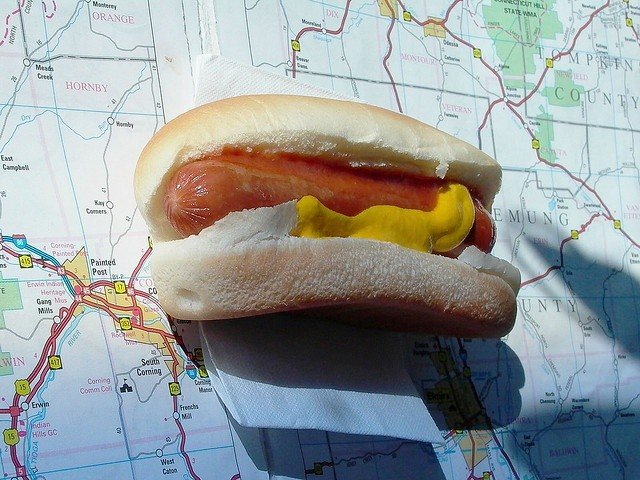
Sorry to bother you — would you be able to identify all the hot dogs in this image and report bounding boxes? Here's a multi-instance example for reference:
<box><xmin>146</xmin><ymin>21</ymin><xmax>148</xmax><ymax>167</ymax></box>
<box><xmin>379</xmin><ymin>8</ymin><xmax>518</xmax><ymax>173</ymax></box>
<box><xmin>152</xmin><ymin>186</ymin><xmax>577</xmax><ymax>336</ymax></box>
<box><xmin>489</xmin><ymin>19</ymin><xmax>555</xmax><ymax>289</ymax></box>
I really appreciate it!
<box><xmin>132</xmin><ymin>93</ymin><xmax>522</xmax><ymax>339</ymax></box>
